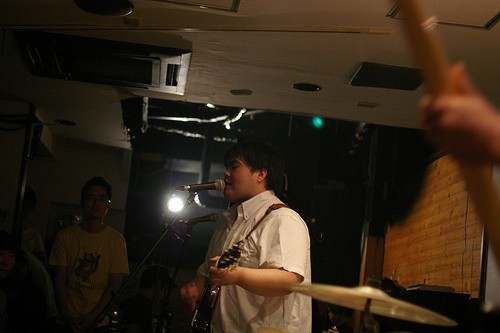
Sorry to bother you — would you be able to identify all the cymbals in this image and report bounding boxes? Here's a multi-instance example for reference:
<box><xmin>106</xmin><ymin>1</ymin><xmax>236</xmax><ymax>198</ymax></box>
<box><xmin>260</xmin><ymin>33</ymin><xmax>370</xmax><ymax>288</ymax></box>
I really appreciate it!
<box><xmin>286</xmin><ymin>282</ymin><xmax>456</xmax><ymax>327</ymax></box>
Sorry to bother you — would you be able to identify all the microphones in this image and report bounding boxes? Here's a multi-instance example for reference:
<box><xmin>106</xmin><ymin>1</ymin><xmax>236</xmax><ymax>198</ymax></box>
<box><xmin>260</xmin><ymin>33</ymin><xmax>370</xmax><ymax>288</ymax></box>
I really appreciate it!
<box><xmin>184</xmin><ymin>212</ymin><xmax>218</xmax><ymax>224</ymax></box>
<box><xmin>175</xmin><ymin>180</ymin><xmax>225</xmax><ymax>191</ymax></box>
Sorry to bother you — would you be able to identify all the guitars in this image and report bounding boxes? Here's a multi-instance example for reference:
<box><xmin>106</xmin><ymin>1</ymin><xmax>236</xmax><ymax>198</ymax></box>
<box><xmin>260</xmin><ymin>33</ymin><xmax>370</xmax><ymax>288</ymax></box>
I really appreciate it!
<box><xmin>178</xmin><ymin>241</ymin><xmax>245</xmax><ymax>333</ymax></box>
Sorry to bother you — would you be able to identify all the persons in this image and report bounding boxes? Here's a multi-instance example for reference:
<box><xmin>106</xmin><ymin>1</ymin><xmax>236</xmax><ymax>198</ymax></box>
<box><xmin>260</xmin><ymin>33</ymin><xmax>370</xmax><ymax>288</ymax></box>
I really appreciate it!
<box><xmin>416</xmin><ymin>60</ymin><xmax>500</xmax><ymax>276</ymax></box>
<box><xmin>178</xmin><ymin>140</ymin><xmax>313</xmax><ymax>333</ymax></box>
<box><xmin>0</xmin><ymin>176</ymin><xmax>170</xmax><ymax>333</ymax></box>
<box><xmin>318</xmin><ymin>302</ymin><xmax>355</xmax><ymax>333</ymax></box>
